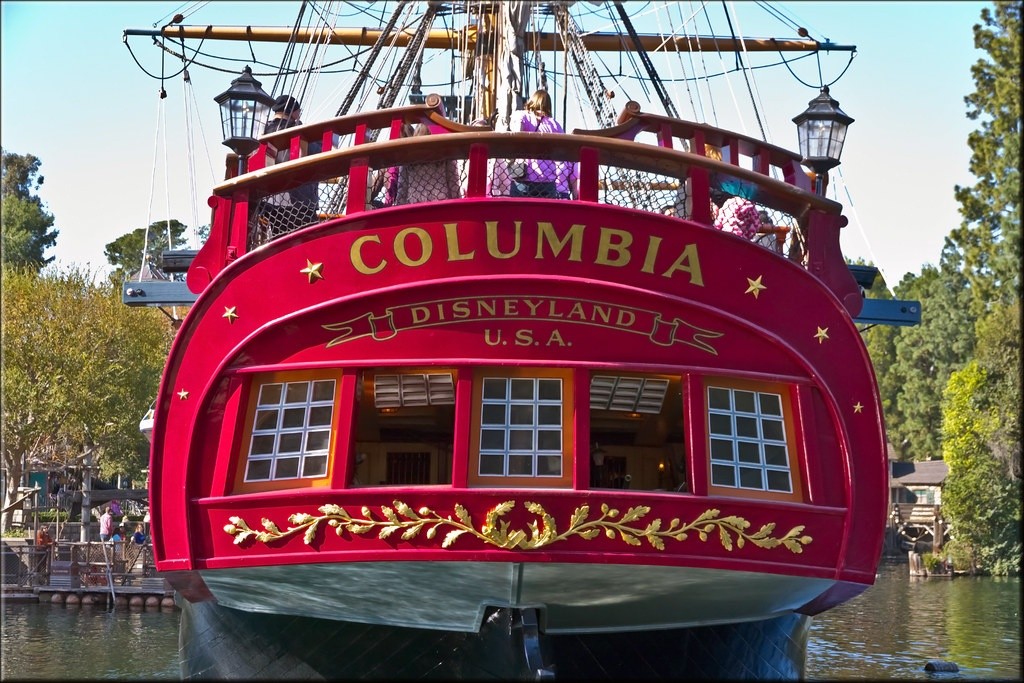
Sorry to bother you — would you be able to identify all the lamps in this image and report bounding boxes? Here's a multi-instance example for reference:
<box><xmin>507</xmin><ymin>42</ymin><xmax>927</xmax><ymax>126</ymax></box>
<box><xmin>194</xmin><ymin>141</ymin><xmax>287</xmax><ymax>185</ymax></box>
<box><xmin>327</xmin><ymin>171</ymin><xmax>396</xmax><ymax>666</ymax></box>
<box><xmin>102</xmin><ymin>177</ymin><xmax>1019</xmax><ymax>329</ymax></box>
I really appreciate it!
<box><xmin>213</xmin><ymin>66</ymin><xmax>277</xmax><ymax>177</ymax></box>
<box><xmin>792</xmin><ymin>85</ymin><xmax>855</xmax><ymax>197</ymax></box>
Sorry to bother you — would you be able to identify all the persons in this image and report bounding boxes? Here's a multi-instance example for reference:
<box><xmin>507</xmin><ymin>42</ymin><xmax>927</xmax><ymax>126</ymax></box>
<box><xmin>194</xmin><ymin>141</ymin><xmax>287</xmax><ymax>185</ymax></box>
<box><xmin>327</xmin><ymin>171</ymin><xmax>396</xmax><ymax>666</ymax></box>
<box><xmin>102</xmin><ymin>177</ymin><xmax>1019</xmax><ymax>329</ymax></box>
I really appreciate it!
<box><xmin>100</xmin><ymin>506</ymin><xmax>143</xmax><ymax>556</ymax></box>
<box><xmin>35</xmin><ymin>525</ymin><xmax>58</xmax><ymax>572</ymax></box>
<box><xmin>661</xmin><ymin>143</ymin><xmax>781</xmax><ymax>255</ymax></box>
<box><xmin>497</xmin><ymin>90</ymin><xmax>577</xmax><ymax>201</ymax></box>
<box><xmin>258</xmin><ymin>96</ymin><xmax>460</xmax><ymax>238</ymax></box>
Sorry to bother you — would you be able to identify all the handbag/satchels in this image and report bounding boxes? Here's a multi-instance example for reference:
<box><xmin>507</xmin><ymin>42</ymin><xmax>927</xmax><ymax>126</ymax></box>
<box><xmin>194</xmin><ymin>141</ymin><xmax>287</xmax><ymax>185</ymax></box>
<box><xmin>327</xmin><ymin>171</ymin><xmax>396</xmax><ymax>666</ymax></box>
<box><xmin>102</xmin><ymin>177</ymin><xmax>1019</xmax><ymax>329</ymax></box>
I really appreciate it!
<box><xmin>373</xmin><ymin>186</ymin><xmax>386</xmax><ymax>208</ymax></box>
<box><xmin>509</xmin><ymin>164</ymin><xmax>526</xmax><ymax>178</ymax></box>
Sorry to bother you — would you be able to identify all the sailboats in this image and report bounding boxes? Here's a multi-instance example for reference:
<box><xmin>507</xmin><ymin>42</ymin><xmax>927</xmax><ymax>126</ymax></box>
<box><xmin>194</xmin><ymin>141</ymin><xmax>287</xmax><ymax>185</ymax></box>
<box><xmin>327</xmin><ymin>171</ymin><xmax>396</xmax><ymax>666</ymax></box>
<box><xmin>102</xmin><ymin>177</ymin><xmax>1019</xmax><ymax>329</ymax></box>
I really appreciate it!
<box><xmin>108</xmin><ymin>3</ymin><xmax>919</xmax><ymax>683</ymax></box>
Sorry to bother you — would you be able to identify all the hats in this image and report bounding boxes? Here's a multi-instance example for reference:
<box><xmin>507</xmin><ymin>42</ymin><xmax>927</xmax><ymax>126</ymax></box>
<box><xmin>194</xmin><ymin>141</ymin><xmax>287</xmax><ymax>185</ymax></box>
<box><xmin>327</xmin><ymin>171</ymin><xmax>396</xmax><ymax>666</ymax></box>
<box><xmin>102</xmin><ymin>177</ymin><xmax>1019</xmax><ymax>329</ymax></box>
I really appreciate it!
<box><xmin>272</xmin><ymin>95</ymin><xmax>300</xmax><ymax>113</ymax></box>
<box><xmin>119</xmin><ymin>522</ymin><xmax>128</xmax><ymax>527</ymax></box>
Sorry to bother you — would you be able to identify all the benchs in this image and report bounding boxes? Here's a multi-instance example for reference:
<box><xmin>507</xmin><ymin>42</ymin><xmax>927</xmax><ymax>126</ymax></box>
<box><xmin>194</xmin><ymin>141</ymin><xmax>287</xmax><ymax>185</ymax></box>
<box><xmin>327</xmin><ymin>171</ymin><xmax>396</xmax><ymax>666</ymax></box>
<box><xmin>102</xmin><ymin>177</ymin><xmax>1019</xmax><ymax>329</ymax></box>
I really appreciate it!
<box><xmin>56</xmin><ymin>542</ymin><xmax>128</xmax><ymax>586</ymax></box>
